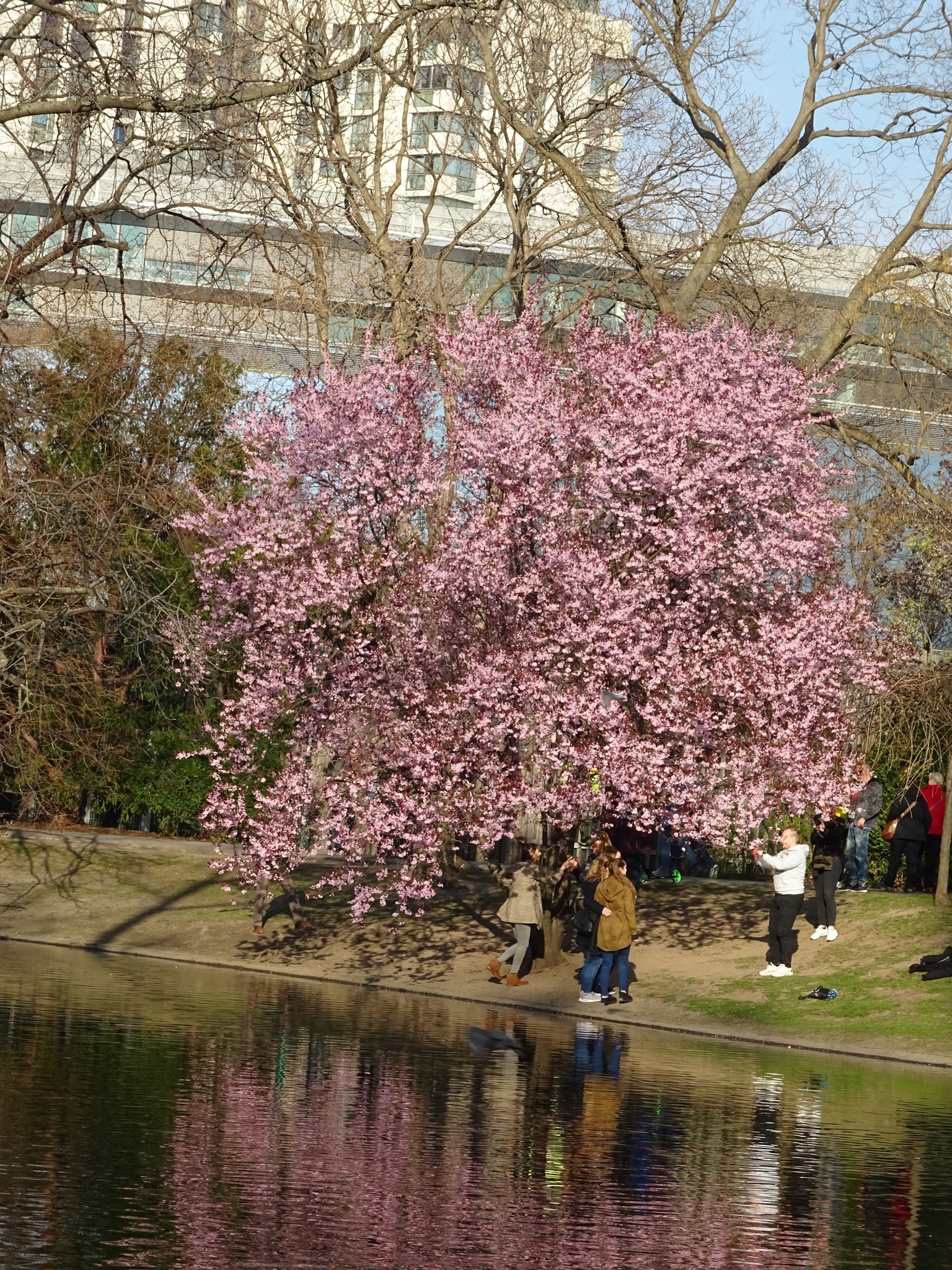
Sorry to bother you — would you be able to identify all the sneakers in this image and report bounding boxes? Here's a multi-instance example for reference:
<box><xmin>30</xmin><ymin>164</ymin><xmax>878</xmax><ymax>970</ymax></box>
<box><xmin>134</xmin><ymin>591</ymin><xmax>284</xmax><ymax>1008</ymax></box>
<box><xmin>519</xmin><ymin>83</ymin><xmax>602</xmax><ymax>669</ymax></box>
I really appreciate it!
<box><xmin>810</xmin><ymin>925</ymin><xmax>828</xmax><ymax>940</ymax></box>
<box><xmin>601</xmin><ymin>995</ymin><xmax>617</xmax><ymax>1006</ymax></box>
<box><xmin>652</xmin><ymin>868</ymin><xmax>668</xmax><ymax>876</ymax></box>
<box><xmin>618</xmin><ymin>991</ymin><xmax>633</xmax><ymax>1002</ymax></box>
<box><xmin>579</xmin><ymin>991</ymin><xmax>601</xmax><ymax>1001</ymax></box>
<box><xmin>690</xmin><ymin>865</ymin><xmax>703</xmax><ymax>877</ymax></box>
<box><xmin>581</xmin><ymin>992</ymin><xmax>601</xmax><ymax>1002</ymax></box>
<box><xmin>849</xmin><ymin>883</ymin><xmax>867</xmax><ymax>893</ymax></box>
<box><xmin>709</xmin><ymin>864</ymin><xmax>719</xmax><ymax>879</ymax></box>
<box><xmin>771</xmin><ymin>963</ymin><xmax>793</xmax><ymax>977</ymax></box>
<box><xmin>837</xmin><ymin>883</ymin><xmax>853</xmax><ymax>891</ymax></box>
<box><xmin>759</xmin><ymin>962</ymin><xmax>779</xmax><ymax>976</ymax></box>
<box><xmin>826</xmin><ymin>927</ymin><xmax>838</xmax><ymax>942</ymax></box>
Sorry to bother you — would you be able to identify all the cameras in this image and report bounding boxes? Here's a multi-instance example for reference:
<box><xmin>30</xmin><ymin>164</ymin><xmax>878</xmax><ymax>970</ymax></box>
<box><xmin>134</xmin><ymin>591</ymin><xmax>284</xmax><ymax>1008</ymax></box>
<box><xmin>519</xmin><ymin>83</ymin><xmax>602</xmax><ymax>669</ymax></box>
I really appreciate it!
<box><xmin>814</xmin><ymin>814</ymin><xmax>823</xmax><ymax>825</ymax></box>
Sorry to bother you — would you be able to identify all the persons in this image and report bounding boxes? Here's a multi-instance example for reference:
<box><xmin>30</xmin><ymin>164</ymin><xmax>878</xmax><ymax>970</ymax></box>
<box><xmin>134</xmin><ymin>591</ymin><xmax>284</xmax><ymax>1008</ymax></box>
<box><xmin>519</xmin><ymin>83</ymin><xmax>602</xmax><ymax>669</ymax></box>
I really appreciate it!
<box><xmin>486</xmin><ymin>844</ymin><xmax>575</xmax><ymax>988</ymax></box>
<box><xmin>330</xmin><ymin>790</ymin><xmax>356</xmax><ymax>854</ymax></box>
<box><xmin>914</xmin><ymin>772</ymin><xmax>946</xmax><ymax>893</ymax></box>
<box><xmin>885</xmin><ymin>774</ymin><xmax>932</xmax><ymax>892</ymax></box>
<box><xmin>652</xmin><ymin>784</ymin><xmax>719</xmax><ymax>878</ymax></box>
<box><xmin>837</xmin><ymin>763</ymin><xmax>882</xmax><ymax>892</ymax></box>
<box><xmin>570</xmin><ymin>831</ymin><xmax>613</xmax><ymax>980</ymax></box>
<box><xmin>809</xmin><ymin>804</ymin><xmax>847</xmax><ymax>941</ymax></box>
<box><xmin>754</xmin><ymin>828</ymin><xmax>809</xmax><ymax>977</ymax></box>
<box><xmin>594</xmin><ymin>858</ymin><xmax>639</xmax><ymax>1006</ymax></box>
<box><xmin>579</xmin><ymin>848</ymin><xmax>621</xmax><ymax>1002</ymax></box>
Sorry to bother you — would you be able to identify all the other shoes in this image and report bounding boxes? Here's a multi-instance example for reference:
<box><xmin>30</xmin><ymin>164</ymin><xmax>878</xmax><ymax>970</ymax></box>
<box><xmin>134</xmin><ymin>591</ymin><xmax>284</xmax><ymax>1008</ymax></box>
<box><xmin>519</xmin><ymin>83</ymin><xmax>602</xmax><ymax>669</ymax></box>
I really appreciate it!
<box><xmin>926</xmin><ymin>887</ymin><xmax>933</xmax><ymax>893</ymax></box>
<box><xmin>608</xmin><ymin>988</ymin><xmax>616</xmax><ymax>994</ymax></box>
<box><xmin>902</xmin><ymin>886</ymin><xmax>916</xmax><ymax>891</ymax></box>
<box><xmin>880</xmin><ymin>879</ymin><xmax>892</xmax><ymax>892</ymax></box>
<box><xmin>903</xmin><ymin>887</ymin><xmax>913</xmax><ymax>893</ymax></box>
<box><xmin>836</xmin><ymin>881</ymin><xmax>841</xmax><ymax>888</ymax></box>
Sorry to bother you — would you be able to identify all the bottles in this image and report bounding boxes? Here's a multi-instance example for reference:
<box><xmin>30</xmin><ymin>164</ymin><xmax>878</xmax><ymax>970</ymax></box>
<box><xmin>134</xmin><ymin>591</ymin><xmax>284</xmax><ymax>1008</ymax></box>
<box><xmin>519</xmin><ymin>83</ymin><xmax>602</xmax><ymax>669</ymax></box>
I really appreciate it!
<box><xmin>827</xmin><ymin>989</ymin><xmax>838</xmax><ymax>999</ymax></box>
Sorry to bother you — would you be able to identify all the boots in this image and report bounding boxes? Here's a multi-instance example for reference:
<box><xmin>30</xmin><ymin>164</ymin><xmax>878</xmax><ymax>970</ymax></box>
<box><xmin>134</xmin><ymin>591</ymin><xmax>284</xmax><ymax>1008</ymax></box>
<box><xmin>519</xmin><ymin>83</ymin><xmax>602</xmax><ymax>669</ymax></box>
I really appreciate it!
<box><xmin>487</xmin><ymin>958</ymin><xmax>502</xmax><ymax>980</ymax></box>
<box><xmin>506</xmin><ymin>973</ymin><xmax>528</xmax><ymax>987</ymax></box>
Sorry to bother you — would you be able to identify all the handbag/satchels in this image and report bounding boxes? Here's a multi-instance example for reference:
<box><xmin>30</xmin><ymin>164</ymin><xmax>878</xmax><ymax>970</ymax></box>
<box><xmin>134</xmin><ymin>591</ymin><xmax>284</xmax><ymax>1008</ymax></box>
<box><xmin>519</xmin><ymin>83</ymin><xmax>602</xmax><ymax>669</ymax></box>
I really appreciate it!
<box><xmin>814</xmin><ymin>855</ymin><xmax>834</xmax><ymax>870</ymax></box>
<box><xmin>881</xmin><ymin>817</ymin><xmax>900</xmax><ymax>841</ymax></box>
<box><xmin>571</xmin><ymin>909</ymin><xmax>593</xmax><ymax>932</ymax></box>
